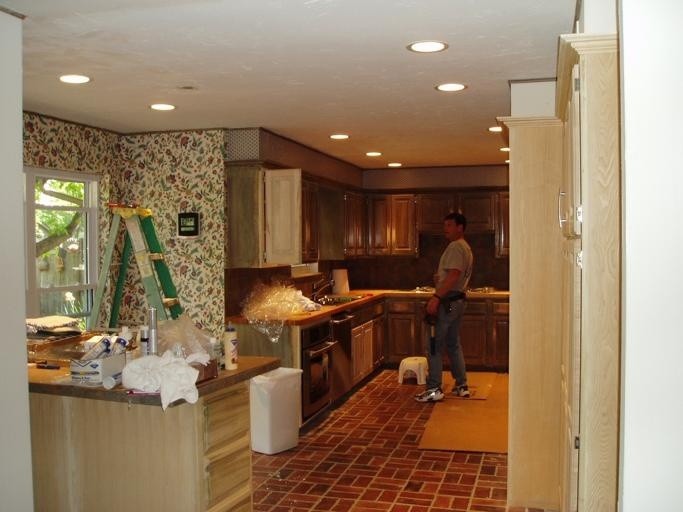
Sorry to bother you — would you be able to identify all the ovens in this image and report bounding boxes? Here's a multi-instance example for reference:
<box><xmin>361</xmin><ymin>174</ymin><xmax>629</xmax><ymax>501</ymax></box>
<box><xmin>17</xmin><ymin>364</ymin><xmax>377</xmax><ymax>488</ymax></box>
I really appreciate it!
<box><xmin>304</xmin><ymin>340</ymin><xmax>339</xmax><ymax>417</ymax></box>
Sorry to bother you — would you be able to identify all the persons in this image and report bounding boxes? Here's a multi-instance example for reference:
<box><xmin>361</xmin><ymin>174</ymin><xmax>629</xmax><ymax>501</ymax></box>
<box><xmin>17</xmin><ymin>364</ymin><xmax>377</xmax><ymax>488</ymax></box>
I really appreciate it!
<box><xmin>412</xmin><ymin>211</ymin><xmax>475</xmax><ymax>403</ymax></box>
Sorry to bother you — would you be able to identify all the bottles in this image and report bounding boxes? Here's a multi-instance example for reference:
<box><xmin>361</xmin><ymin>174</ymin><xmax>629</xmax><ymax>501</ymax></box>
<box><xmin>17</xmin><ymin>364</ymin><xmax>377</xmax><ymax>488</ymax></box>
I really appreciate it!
<box><xmin>223</xmin><ymin>321</ymin><xmax>239</xmax><ymax>370</ymax></box>
<box><xmin>139</xmin><ymin>305</ymin><xmax>158</xmax><ymax>356</ymax></box>
<box><xmin>80</xmin><ymin>336</ymin><xmax>129</xmax><ymax>362</ymax></box>
<box><xmin>102</xmin><ymin>371</ymin><xmax>121</xmax><ymax>390</ymax></box>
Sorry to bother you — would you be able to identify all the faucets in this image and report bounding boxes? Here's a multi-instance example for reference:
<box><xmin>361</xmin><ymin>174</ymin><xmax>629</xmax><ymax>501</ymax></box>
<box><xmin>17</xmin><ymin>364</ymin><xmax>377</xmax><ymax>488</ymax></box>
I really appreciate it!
<box><xmin>313</xmin><ymin>276</ymin><xmax>335</xmax><ymax>303</ymax></box>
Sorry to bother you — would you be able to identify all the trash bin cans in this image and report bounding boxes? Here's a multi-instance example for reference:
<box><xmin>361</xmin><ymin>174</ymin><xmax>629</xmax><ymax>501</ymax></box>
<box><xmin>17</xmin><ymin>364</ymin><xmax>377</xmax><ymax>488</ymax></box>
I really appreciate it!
<box><xmin>250</xmin><ymin>367</ymin><xmax>304</xmax><ymax>455</ymax></box>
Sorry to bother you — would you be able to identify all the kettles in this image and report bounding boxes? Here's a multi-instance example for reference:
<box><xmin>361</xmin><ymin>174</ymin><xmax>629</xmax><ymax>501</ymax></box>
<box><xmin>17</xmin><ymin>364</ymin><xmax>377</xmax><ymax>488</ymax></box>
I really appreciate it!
<box><xmin>330</xmin><ymin>269</ymin><xmax>349</xmax><ymax>294</ymax></box>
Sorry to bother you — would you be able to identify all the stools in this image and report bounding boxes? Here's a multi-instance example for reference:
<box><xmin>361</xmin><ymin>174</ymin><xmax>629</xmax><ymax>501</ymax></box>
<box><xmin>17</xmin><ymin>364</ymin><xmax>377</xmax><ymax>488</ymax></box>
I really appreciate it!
<box><xmin>397</xmin><ymin>357</ymin><xmax>427</xmax><ymax>384</ymax></box>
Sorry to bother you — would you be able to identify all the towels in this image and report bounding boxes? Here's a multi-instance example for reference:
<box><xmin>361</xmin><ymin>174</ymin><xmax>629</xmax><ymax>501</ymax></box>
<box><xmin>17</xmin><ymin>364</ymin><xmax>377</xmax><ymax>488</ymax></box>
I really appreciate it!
<box><xmin>121</xmin><ymin>355</ymin><xmax>199</xmax><ymax>412</ymax></box>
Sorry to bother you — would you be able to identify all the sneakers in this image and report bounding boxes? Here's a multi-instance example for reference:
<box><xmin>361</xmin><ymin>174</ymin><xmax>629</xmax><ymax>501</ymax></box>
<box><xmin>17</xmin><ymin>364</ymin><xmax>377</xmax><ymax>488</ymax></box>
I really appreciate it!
<box><xmin>415</xmin><ymin>387</ymin><xmax>444</xmax><ymax>402</ymax></box>
<box><xmin>450</xmin><ymin>383</ymin><xmax>469</xmax><ymax>398</ymax></box>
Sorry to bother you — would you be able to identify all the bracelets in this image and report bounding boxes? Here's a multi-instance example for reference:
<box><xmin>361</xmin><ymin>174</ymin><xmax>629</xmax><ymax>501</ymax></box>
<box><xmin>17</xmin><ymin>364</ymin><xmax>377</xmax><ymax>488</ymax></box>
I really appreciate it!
<box><xmin>432</xmin><ymin>293</ymin><xmax>441</xmax><ymax>301</ymax></box>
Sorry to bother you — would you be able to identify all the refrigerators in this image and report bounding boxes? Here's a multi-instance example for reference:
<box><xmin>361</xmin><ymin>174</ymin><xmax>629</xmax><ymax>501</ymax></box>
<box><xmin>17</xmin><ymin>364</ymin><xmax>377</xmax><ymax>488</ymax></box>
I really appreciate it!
<box><xmin>330</xmin><ymin>314</ymin><xmax>353</xmax><ymax>402</ymax></box>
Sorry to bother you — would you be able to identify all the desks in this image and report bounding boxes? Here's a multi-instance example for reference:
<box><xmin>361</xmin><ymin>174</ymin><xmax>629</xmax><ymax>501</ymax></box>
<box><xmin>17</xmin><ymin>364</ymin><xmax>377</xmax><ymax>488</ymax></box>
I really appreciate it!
<box><xmin>27</xmin><ymin>356</ymin><xmax>283</xmax><ymax>512</ymax></box>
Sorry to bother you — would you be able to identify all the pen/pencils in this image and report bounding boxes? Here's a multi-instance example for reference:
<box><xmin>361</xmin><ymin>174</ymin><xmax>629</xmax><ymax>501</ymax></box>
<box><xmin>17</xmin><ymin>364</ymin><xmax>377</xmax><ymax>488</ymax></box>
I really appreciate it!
<box><xmin>36</xmin><ymin>364</ymin><xmax>60</xmax><ymax>369</ymax></box>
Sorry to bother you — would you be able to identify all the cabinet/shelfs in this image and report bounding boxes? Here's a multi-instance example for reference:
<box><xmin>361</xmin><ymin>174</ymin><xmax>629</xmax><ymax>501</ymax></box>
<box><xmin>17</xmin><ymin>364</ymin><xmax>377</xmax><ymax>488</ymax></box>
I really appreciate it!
<box><xmin>296</xmin><ymin>299</ymin><xmax>388</xmax><ymax>428</ymax></box>
<box><xmin>367</xmin><ymin>191</ymin><xmax>419</xmax><ymax>256</ymax></box>
<box><xmin>494</xmin><ymin>191</ymin><xmax>508</xmax><ymax>261</ymax></box>
<box><xmin>266</xmin><ymin>167</ymin><xmax>365</xmax><ymax>268</ymax></box>
<box><xmin>419</xmin><ymin>192</ymin><xmax>494</xmax><ymax>235</ymax></box>
<box><xmin>384</xmin><ymin>294</ymin><xmax>509</xmax><ymax>367</ymax></box>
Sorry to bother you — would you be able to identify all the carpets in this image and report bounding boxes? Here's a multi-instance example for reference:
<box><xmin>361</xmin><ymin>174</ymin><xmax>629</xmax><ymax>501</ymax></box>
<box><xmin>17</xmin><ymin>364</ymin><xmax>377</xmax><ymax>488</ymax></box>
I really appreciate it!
<box><xmin>417</xmin><ymin>371</ymin><xmax>509</xmax><ymax>454</ymax></box>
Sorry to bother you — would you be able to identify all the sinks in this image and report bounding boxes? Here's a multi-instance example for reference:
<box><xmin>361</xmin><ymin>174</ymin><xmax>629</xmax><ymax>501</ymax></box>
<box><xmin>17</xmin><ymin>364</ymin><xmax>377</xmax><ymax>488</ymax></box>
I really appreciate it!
<box><xmin>317</xmin><ymin>295</ymin><xmax>363</xmax><ymax>304</ymax></box>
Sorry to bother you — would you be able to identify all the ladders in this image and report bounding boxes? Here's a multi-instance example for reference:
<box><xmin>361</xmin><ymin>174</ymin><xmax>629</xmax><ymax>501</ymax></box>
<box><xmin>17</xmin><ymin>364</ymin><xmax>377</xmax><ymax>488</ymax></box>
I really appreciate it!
<box><xmin>88</xmin><ymin>204</ymin><xmax>182</xmax><ymax>331</ymax></box>
